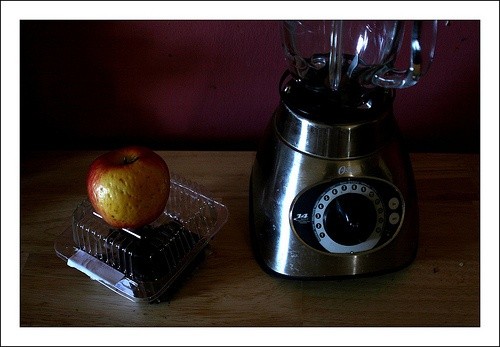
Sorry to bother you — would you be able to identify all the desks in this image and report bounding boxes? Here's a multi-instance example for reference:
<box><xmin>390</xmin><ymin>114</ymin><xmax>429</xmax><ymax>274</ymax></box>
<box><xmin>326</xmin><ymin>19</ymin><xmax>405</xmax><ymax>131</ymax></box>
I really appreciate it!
<box><xmin>20</xmin><ymin>148</ymin><xmax>480</xmax><ymax>327</ymax></box>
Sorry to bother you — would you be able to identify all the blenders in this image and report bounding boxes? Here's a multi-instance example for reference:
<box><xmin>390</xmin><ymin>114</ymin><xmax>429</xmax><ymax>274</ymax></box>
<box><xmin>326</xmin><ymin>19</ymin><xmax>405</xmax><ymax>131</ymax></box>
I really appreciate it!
<box><xmin>247</xmin><ymin>20</ymin><xmax>439</xmax><ymax>282</ymax></box>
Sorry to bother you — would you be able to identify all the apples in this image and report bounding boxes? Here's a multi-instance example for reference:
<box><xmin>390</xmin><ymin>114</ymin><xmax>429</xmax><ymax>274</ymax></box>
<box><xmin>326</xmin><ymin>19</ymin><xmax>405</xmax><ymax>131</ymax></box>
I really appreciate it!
<box><xmin>88</xmin><ymin>146</ymin><xmax>170</xmax><ymax>227</ymax></box>
